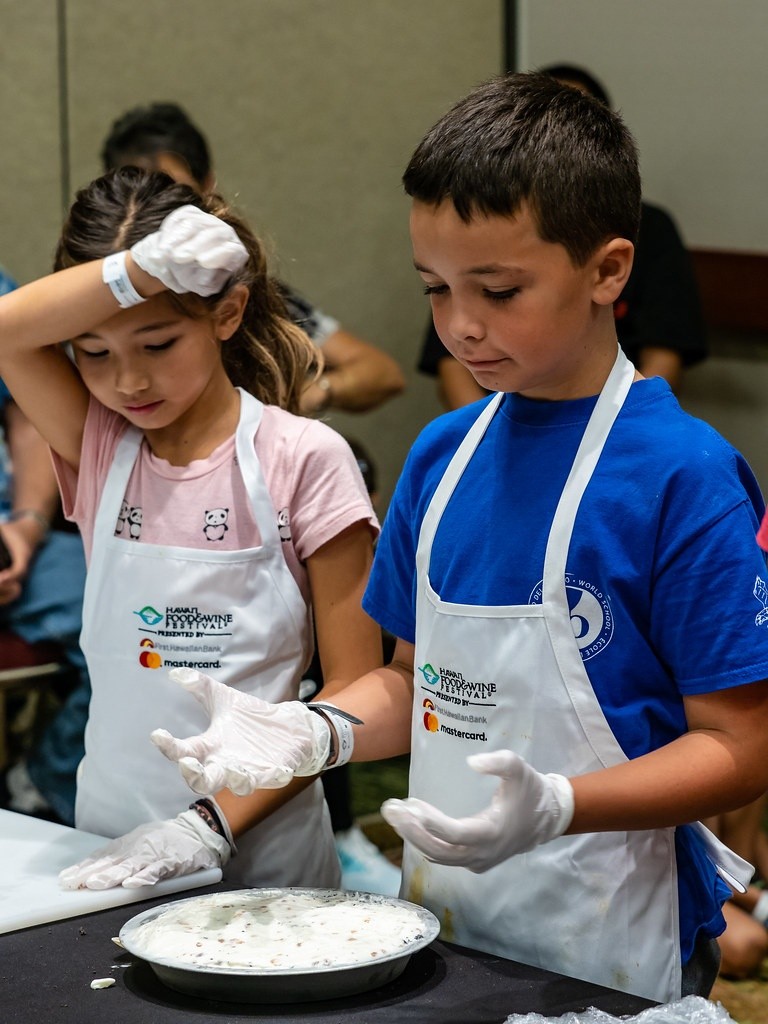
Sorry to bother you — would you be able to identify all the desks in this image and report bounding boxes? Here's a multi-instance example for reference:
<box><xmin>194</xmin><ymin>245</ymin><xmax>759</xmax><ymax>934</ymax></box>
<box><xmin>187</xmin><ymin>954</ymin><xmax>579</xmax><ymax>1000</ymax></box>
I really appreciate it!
<box><xmin>0</xmin><ymin>805</ymin><xmax>741</xmax><ymax>1024</ymax></box>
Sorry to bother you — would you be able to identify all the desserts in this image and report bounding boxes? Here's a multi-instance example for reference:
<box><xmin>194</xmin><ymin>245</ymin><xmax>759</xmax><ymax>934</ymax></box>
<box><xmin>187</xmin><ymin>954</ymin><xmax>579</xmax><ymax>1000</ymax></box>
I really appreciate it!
<box><xmin>118</xmin><ymin>886</ymin><xmax>440</xmax><ymax>1003</ymax></box>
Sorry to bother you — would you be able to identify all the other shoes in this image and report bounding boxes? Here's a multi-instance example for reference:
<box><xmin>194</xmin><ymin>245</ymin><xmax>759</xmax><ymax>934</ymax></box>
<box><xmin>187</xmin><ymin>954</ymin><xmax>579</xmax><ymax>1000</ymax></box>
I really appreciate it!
<box><xmin>5</xmin><ymin>761</ymin><xmax>51</xmax><ymax>812</ymax></box>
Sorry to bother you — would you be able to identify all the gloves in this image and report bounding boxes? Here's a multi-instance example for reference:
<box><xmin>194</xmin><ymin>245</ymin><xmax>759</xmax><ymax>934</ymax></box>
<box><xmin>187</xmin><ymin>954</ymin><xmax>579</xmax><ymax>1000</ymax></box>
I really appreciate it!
<box><xmin>378</xmin><ymin>749</ymin><xmax>576</xmax><ymax>874</ymax></box>
<box><xmin>129</xmin><ymin>204</ymin><xmax>250</xmax><ymax>297</ymax></box>
<box><xmin>57</xmin><ymin>796</ymin><xmax>238</xmax><ymax>889</ymax></box>
<box><xmin>148</xmin><ymin>666</ymin><xmax>332</xmax><ymax>796</ymax></box>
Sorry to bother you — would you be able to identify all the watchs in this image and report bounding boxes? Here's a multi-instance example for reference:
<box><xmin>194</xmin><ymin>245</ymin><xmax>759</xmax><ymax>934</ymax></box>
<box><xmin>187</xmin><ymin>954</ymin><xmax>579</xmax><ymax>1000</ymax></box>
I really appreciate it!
<box><xmin>315</xmin><ymin>378</ymin><xmax>333</xmax><ymax>412</ymax></box>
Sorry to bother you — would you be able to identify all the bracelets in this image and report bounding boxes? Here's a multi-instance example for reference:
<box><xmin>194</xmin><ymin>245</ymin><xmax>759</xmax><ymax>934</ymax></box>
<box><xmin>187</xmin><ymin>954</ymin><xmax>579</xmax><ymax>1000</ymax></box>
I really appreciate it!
<box><xmin>103</xmin><ymin>249</ymin><xmax>147</xmax><ymax>308</ymax></box>
<box><xmin>10</xmin><ymin>509</ymin><xmax>50</xmax><ymax>533</ymax></box>
<box><xmin>305</xmin><ymin>701</ymin><xmax>365</xmax><ymax>770</ymax></box>
<box><xmin>189</xmin><ymin>795</ymin><xmax>239</xmax><ymax>857</ymax></box>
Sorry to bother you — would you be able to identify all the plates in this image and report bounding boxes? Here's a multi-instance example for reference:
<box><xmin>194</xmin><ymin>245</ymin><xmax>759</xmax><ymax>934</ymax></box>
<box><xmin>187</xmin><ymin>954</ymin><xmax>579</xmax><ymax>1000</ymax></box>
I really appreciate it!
<box><xmin>119</xmin><ymin>886</ymin><xmax>441</xmax><ymax>994</ymax></box>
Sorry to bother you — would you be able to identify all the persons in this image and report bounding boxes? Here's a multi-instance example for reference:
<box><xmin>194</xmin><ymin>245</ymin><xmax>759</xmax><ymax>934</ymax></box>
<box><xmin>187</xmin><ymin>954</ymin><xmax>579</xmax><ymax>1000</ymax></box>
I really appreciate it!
<box><xmin>150</xmin><ymin>76</ymin><xmax>768</xmax><ymax>1005</ymax></box>
<box><xmin>416</xmin><ymin>63</ymin><xmax>708</xmax><ymax>412</ymax></box>
<box><xmin>103</xmin><ymin>101</ymin><xmax>406</xmax><ymax>415</ymax></box>
<box><xmin>0</xmin><ymin>163</ymin><xmax>381</xmax><ymax>890</ymax></box>
<box><xmin>0</xmin><ymin>271</ymin><xmax>88</xmax><ymax>825</ymax></box>
<box><xmin>702</xmin><ymin>794</ymin><xmax>768</xmax><ymax>982</ymax></box>
